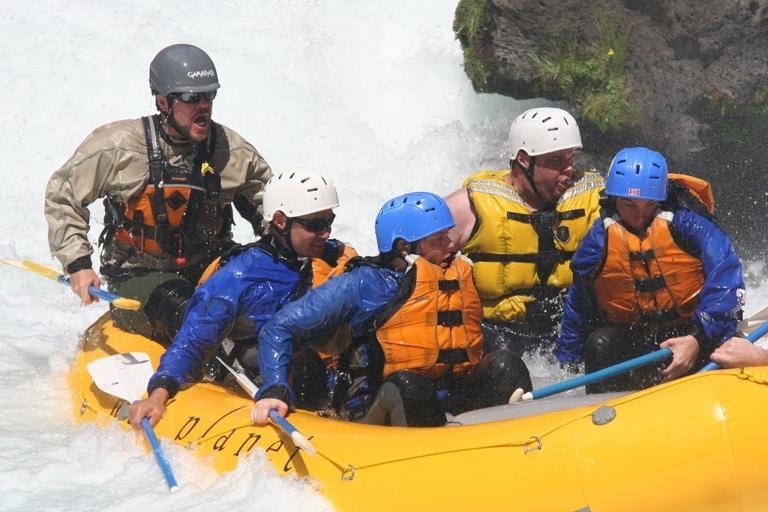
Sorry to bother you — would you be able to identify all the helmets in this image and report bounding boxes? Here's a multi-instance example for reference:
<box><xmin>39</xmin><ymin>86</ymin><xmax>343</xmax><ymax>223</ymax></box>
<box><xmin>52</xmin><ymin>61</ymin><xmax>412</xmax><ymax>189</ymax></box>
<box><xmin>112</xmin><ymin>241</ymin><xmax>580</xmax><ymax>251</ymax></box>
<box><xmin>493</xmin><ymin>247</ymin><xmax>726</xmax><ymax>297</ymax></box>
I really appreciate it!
<box><xmin>262</xmin><ymin>167</ymin><xmax>339</xmax><ymax>223</ymax></box>
<box><xmin>149</xmin><ymin>44</ymin><xmax>220</xmax><ymax>99</ymax></box>
<box><xmin>605</xmin><ymin>146</ymin><xmax>669</xmax><ymax>202</ymax></box>
<box><xmin>509</xmin><ymin>106</ymin><xmax>582</xmax><ymax>161</ymax></box>
<box><xmin>375</xmin><ymin>192</ymin><xmax>456</xmax><ymax>253</ymax></box>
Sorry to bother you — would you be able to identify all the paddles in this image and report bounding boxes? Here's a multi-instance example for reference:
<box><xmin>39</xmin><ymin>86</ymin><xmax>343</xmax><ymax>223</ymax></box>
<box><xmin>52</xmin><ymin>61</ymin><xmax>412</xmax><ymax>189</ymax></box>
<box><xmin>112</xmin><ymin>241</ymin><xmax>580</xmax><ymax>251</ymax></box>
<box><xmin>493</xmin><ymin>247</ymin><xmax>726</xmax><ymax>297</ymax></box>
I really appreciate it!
<box><xmin>8</xmin><ymin>258</ymin><xmax>140</xmax><ymax>309</ymax></box>
<box><xmin>87</xmin><ymin>352</ymin><xmax>182</xmax><ymax>494</ymax></box>
<box><xmin>206</xmin><ymin>336</ymin><xmax>313</xmax><ymax>453</ymax></box>
<box><xmin>508</xmin><ymin>305</ymin><xmax>766</xmax><ymax>405</ymax></box>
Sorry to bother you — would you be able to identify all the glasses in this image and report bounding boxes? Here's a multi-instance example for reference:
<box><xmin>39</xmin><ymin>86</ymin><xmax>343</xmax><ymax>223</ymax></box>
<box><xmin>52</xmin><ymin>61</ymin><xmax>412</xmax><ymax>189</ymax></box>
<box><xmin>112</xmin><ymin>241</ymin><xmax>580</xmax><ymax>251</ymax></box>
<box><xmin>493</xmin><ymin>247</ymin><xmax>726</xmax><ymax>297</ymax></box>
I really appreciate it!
<box><xmin>293</xmin><ymin>214</ymin><xmax>336</xmax><ymax>230</ymax></box>
<box><xmin>177</xmin><ymin>90</ymin><xmax>216</xmax><ymax>103</ymax></box>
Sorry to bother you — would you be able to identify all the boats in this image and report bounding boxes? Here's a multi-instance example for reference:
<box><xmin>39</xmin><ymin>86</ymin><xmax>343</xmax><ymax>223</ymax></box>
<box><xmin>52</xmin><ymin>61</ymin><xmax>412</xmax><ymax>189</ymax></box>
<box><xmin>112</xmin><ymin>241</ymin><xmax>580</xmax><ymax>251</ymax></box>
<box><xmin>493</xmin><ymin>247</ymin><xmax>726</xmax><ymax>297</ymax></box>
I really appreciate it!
<box><xmin>67</xmin><ymin>308</ymin><xmax>767</xmax><ymax>511</ymax></box>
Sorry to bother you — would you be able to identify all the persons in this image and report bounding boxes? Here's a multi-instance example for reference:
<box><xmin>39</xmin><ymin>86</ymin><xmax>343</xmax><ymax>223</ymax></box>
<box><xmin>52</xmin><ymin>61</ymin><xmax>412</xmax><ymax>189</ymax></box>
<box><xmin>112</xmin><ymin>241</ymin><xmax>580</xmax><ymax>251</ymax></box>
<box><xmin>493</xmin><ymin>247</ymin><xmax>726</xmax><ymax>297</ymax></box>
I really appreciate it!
<box><xmin>42</xmin><ymin>41</ymin><xmax>275</xmax><ymax>360</ymax></box>
<box><xmin>552</xmin><ymin>143</ymin><xmax>747</xmax><ymax>397</ymax></box>
<box><xmin>707</xmin><ymin>334</ymin><xmax>768</xmax><ymax>371</ymax></box>
<box><xmin>439</xmin><ymin>104</ymin><xmax>609</xmax><ymax>362</ymax></box>
<box><xmin>126</xmin><ymin>167</ymin><xmax>361</xmax><ymax>430</ymax></box>
<box><xmin>246</xmin><ymin>190</ymin><xmax>535</xmax><ymax>430</ymax></box>
